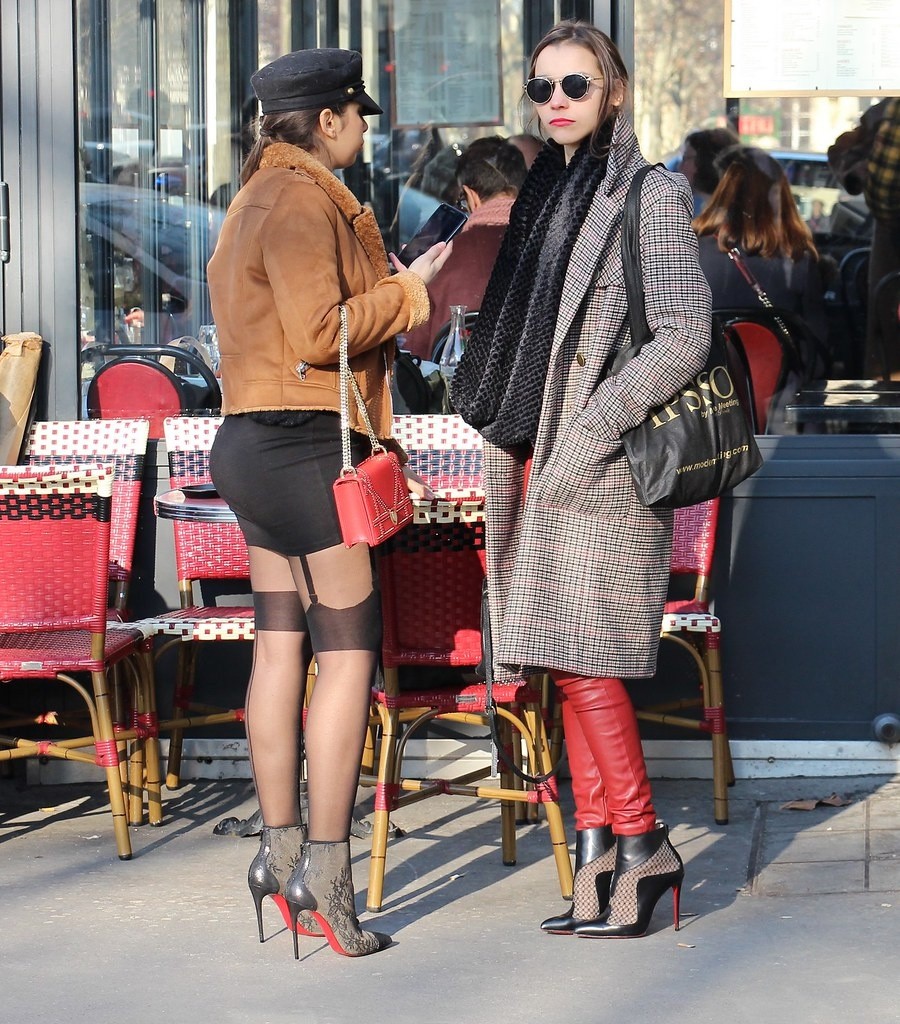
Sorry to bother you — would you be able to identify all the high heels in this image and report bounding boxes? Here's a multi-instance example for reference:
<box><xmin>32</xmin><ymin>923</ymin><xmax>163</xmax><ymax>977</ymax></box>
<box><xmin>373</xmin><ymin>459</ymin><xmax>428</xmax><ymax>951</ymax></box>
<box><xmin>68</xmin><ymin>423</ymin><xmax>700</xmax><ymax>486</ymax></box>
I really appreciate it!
<box><xmin>574</xmin><ymin>822</ymin><xmax>684</xmax><ymax>939</ymax></box>
<box><xmin>248</xmin><ymin>826</ymin><xmax>359</xmax><ymax>944</ymax></box>
<box><xmin>540</xmin><ymin>824</ymin><xmax>618</xmax><ymax>935</ymax></box>
<box><xmin>285</xmin><ymin>841</ymin><xmax>392</xmax><ymax>961</ymax></box>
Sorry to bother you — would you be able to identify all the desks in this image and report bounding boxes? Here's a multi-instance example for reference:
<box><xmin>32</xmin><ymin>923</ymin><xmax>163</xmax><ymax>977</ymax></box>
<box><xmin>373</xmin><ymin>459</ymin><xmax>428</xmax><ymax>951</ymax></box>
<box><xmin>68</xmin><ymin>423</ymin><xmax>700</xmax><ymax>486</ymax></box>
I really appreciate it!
<box><xmin>785</xmin><ymin>379</ymin><xmax>900</xmax><ymax>434</ymax></box>
<box><xmin>153</xmin><ymin>488</ymin><xmax>404</xmax><ymax>841</ymax></box>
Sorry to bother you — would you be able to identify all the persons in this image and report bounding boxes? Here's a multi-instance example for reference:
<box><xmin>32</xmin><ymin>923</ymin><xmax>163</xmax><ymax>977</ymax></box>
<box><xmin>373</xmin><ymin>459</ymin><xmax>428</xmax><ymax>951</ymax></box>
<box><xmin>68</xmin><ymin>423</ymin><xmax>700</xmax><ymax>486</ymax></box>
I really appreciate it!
<box><xmin>208</xmin><ymin>49</ymin><xmax>454</xmax><ymax>955</ymax></box>
<box><xmin>690</xmin><ymin>145</ymin><xmax>849</xmax><ymax>376</ymax></box>
<box><xmin>828</xmin><ymin>97</ymin><xmax>900</xmax><ymax>376</ymax></box>
<box><xmin>448</xmin><ymin>22</ymin><xmax>713</xmax><ymax>939</ymax></box>
<box><xmin>508</xmin><ymin>133</ymin><xmax>544</xmax><ymax>169</ymax></box>
<box><xmin>678</xmin><ymin>126</ymin><xmax>742</xmax><ymax>209</ymax></box>
<box><xmin>401</xmin><ymin>134</ymin><xmax>528</xmax><ymax>360</ymax></box>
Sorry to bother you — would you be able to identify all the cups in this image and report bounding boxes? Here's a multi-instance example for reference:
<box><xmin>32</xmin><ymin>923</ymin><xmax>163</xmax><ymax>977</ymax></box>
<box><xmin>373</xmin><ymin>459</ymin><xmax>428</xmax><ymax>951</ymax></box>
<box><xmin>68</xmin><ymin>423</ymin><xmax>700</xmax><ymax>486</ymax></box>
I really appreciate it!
<box><xmin>201</xmin><ymin>324</ymin><xmax>220</xmax><ymax>375</ymax></box>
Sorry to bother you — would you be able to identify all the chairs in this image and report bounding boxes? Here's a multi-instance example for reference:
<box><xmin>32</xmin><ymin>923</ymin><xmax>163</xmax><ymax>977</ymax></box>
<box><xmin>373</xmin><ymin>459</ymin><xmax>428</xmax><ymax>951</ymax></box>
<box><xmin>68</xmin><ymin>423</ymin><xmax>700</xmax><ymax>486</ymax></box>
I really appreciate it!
<box><xmin>712</xmin><ymin>306</ymin><xmax>817</xmax><ymax>436</ymax></box>
<box><xmin>81</xmin><ymin>344</ymin><xmax>222</xmax><ymax>439</ymax></box>
<box><xmin>0</xmin><ymin>463</ymin><xmax>134</xmax><ymax>860</ymax></box>
<box><xmin>362</xmin><ymin>415</ymin><xmax>572</xmax><ymax>913</ymax></box>
<box><xmin>549</xmin><ymin>496</ymin><xmax>736</xmax><ymax>826</ymax></box>
<box><xmin>136</xmin><ymin>415</ymin><xmax>307</xmax><ymax>826</ymax></box>
<box><xmin>16</xmin><ymin>419</ymin><xmax>150</xmax><ymax>825</ymax></box>
<box><xmin>838</xmin><ymin>247</ymin><xmax>871</xmax><ymax>378</ymax></box>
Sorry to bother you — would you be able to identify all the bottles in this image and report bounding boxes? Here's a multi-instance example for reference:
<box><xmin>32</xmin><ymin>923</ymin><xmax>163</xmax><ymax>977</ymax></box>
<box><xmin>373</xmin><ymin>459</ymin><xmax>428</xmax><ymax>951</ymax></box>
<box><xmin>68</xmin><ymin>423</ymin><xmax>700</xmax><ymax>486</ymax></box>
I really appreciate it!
<box><xmin>162</xmin><ymin>294</ymin><xmax>177</xmax><ymax>340</ymax></box>
<box><xmin>439</xmin><ymin>305</ymin><xmax>468</xmax><ymax>407</ymax></box>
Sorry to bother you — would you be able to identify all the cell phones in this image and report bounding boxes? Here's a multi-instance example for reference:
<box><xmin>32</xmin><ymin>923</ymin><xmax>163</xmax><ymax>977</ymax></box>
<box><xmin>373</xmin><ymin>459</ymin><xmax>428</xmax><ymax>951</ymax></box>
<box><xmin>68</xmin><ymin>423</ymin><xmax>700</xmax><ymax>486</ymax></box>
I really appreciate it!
<box><xmin>397</xmin><ymin>203</ymin><xmax>468</xmax><ymax>269</ymax></box>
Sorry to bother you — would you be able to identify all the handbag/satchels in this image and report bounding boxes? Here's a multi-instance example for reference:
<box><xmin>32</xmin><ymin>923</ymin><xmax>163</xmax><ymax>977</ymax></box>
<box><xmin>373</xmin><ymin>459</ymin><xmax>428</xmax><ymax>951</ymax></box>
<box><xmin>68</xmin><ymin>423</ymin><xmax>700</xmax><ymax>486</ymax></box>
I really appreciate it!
<box><xmin>334</xmin><ymin>306</ymin><xmax>413</xmax><ymax>548</ymax></box>
<box><xmin>604</xmin><ymin>326</ymin><xmax>763</xmax><ymax>510</ymax></box>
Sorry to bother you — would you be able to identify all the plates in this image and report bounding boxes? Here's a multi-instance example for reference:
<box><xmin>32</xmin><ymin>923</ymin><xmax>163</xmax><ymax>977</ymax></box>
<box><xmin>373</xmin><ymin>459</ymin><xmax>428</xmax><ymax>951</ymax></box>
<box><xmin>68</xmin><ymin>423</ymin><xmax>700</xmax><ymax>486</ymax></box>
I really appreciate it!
<box><xmin>179</xmin><ymin>484</ymin><xmax>220</xmax><ymax>500</ymax></box>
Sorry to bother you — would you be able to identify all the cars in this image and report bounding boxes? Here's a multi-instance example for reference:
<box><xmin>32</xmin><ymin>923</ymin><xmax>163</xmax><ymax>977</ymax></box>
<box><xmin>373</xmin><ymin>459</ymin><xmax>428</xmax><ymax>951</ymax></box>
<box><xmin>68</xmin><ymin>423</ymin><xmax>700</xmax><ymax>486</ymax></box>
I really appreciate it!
<box><xmin>79</xmin><ymin>184</ymin><xmax>231</xmax><ymax>365</ymax></box>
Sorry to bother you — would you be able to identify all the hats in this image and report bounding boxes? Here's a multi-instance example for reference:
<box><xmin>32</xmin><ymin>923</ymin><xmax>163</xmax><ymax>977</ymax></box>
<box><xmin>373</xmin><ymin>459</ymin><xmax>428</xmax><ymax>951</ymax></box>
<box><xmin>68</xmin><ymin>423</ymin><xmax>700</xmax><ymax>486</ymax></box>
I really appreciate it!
<box><xmin>251</xmin><ymin>49</ymin><xmax>383</xmax><ymax>117</ymax></box>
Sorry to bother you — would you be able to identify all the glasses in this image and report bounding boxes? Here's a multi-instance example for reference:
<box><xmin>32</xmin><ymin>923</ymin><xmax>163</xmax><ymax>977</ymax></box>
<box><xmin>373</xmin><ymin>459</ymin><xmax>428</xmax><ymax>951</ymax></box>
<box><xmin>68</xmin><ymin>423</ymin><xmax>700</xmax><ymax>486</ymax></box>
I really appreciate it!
<box><xmin>454</xmin><ymin>191</ymin><xmax>469</xmax><ymax>212</ymax></box>
<box><xmin>522</xmin><ymin>73</ymin><xmax>602</xmax><ymax>105</ymax></box>
<box><xmin>682</xmin><ymin>151</ymin><xmax>697</xmax><ymax>163</ymax></box>
